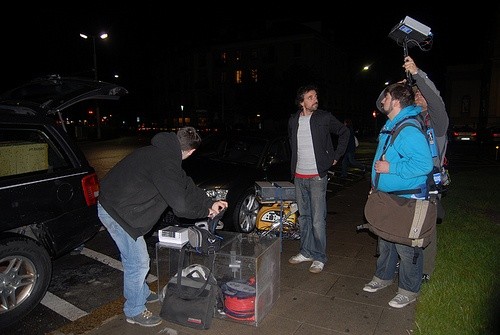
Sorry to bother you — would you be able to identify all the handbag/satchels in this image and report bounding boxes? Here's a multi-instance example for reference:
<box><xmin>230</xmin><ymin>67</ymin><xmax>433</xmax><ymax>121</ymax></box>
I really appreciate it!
<box><xmin>159</xmin><ymin>264</ymin><xmax>218</xmax><ymax>330</ymax></box>
<box><xmin>356</xmin><ymin>188</ymin><xmax>438</xmax><ymax>264</ymax></box>
<box><xmin>354</xmin><ymin>135</ymin><xmax>359</xmax><ymax>147</ymax></box>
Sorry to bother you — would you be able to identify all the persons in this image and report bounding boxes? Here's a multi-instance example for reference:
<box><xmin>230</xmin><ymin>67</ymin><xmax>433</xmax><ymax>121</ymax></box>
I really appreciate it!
<box><xmin>363</xmin><ymin>56</ymin><xmax>449</xmax><ymax>310</ymax></box>
<box><xmin>97</xmin><ymin>127</ymin><xmax>228</xmax><ymax>327</ymax></box>
<box><xmin>286</xmin><ymin>85</ymin><xmax>349</xmax><ymax>272</ymax></box>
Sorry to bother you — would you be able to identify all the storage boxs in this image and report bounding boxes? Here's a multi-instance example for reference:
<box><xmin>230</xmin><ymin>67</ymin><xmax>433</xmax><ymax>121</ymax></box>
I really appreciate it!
<box><xmin>156</xmin><ymin>230</ymin><xmax>281</xmax><ymax>327</ymax></box>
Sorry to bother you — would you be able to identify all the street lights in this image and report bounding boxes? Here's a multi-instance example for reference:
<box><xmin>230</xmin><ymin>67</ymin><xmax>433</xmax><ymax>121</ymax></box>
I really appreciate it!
<box><xmin>79</xmin><ymin>31</ymin><xmax>110</xmax><ymax>139</ymax></box>
<box><xmin>221</xmin><ymin>51</ymin><xmax>241</xmax><ymax>122</ymax></box>
<box><xmin>348</xmin><ymin>63</ymin><xmax>373</xmax><ymax>117</ymax></box>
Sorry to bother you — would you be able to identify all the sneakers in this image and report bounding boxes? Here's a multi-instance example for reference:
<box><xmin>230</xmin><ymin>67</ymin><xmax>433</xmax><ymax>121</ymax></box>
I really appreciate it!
<box><xmin>126</xmin><ymin>308</ymin><xmax>163</xmax><ymax>327</ymax></box>
<box><xmin>362</xmin><ymin>280</ymin><xmax>390</xmax><ymax>293</ymax></box>
<box><xmin>309</xmin><ymin>260</ymin><xmax>324</xmax><ymax>273</ymax></box>
<box><xmin>145</xmin><ymin>290</ymin><xmax>162</xmax><ymax>303</ymax></box>
<box><xmin>388</xmin><ymin>293</ymin><xmax>417</xmax><ymax>308</ymax></box>
<box><xmin>288</xmin><ymin>253</ymin><xmax>314</xmax><ymax>263</ymax></box>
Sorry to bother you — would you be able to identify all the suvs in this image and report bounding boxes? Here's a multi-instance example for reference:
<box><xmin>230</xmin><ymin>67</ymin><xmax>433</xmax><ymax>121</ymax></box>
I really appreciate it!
<box><xmin>0</xmin><ymin>76</ymin><xmax>128</xmax><ymax>335</ymax></box>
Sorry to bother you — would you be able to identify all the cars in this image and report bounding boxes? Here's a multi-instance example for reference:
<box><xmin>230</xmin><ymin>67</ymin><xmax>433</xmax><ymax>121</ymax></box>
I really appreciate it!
<box><xmin>449</xmin><ymin>125</ymin><xmax>477</xmax><ymax>143</ymax></box>
<box><xmin>478</xmin><ymin>127</ymin><xmax>500</xmax><ymax>144</ymax></box>
<box><xmin>143</xmin><ymin>112</ymin><xmax>357</xmax><ymax>233</ymax></box>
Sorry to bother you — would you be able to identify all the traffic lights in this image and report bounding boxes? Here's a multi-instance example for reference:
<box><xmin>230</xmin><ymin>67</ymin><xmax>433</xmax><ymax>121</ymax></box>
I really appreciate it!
<box><xmin>372</xmin><ymin>111</ymin><xmax>378</xmax><ymax>118</ymax></box>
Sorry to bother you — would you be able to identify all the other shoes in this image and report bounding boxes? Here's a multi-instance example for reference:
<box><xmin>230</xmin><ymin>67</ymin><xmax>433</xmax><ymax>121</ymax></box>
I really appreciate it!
<box><xmin>421</xmin><ymin>273</ymin><xmax>430</xmax><ymax>284</ymax></box>
<box><xmin>394</xmin><ymin>262</ymin><xmax>400</xmax><ymax>273</ymax></box>
